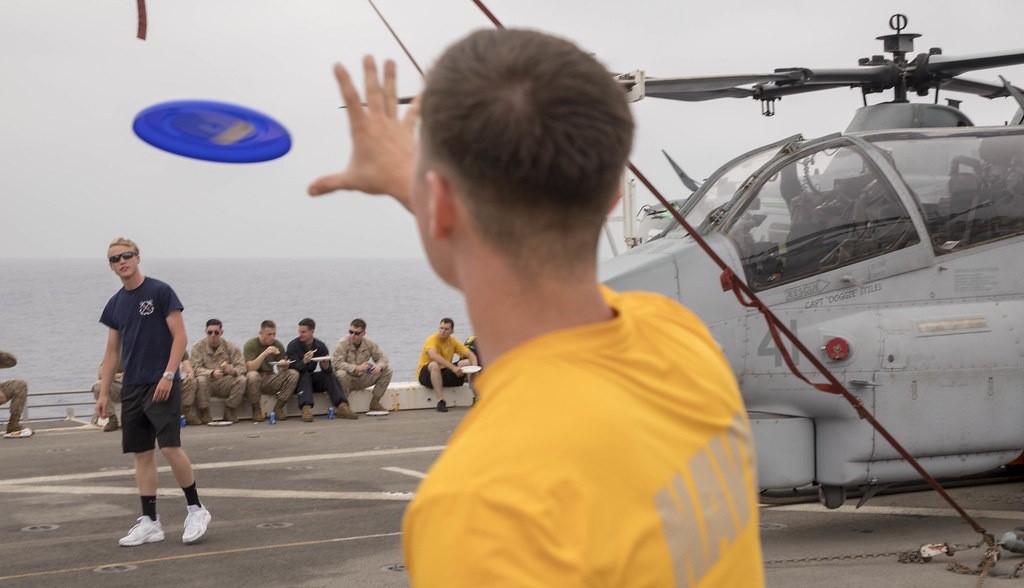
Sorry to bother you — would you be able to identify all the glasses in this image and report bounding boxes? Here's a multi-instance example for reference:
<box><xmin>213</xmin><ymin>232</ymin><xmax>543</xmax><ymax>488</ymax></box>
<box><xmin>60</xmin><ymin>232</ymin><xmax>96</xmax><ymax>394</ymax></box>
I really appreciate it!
<box><xmin>109</xmin><ymin>252</ymin><xmax>137</xmax><ymax>262</ymax></box>
<box><xmin>207</xmin><ymin>330</ymin><xmax>220</xmax><ymax>335</ymax></box>
<box><xmin>349</xmin><ymin>330</ymin><xmax>364</xmax><ymax>335</ymax></box>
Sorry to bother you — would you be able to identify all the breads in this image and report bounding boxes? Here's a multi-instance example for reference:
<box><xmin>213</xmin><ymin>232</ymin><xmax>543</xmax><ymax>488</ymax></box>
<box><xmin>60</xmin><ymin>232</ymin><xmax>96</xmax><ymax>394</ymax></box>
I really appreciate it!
<box><xmin>273</xmin><ymin>348</ymin><xmax>290</xmax><ymax>362</ymax></box>
<box><xmin>220</xmin><ymin>361</ymin><xmax>228</xmax><ymax>369</ymax></box>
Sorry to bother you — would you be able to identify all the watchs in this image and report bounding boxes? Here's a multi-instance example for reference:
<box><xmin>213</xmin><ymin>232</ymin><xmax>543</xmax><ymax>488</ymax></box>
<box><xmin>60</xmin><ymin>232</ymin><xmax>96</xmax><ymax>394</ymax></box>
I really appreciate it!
<box><xmin>163</xmin><ymin>372</ymin><xmax>174</xmax><ymax>379</ymax></box>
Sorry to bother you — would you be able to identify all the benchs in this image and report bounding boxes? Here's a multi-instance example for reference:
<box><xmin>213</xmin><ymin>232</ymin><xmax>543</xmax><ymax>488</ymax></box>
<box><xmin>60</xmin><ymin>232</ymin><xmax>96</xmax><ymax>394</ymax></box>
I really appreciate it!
<box><xmin>111</xmin><ymin>382</ymin><xmax>474</xmax><ymax>425</ymax></box>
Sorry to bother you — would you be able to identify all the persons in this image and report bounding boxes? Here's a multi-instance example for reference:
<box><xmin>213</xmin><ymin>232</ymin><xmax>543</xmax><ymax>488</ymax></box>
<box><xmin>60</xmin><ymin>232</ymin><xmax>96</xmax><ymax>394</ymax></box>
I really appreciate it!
<box><xmin>417</xmin><ymin>318</ymin><xmax>477</xmax><ymax>412</ymax></box>
<box><xmin>460</xmin><ymin>336</ymin><xmax>477</xmax><ymax>360</ymax></box>
<box><xmin>244</xmin><ymin>319</ymin><xmax>299</xmax><ymax>421</ymax></box>
<box><xmin>94</xmin><ymin>237</ymin><xmax>211</xmax><ymax>545</ymax></box>
<box><xmin>332</xmin><ymin>319</ymin><xmax>393</xmax><ymax>412</ymax></box>
<box><xmin>180</xmin><ymin>351</ymin><xmax>202</xmax><ymax>425</ymax></box>
<box><xmin>0</xmin><ymin>352</ymin><xmax>33</xmax><ymax>438</ymax></box>
<box><xmin>754</xmin><ymin>156</ymin><xmax>846</xmax><ymax>284</ymax></box>
<box><xmin>92</xmin><ymin>359</ymin><xmax>123</xmax><ymax>432</ymax></box>
<box><xmin>309</xmin><ymin>28</ymin><xmax>766</xmax><ymax>588</ymax></box>
<box><xmin>286</xmin><ymin>318</ymin><xmax>359</xmax><ymax>422</ymax></box>
<box><xmin>190</xmin><ymin>319</ymin><xmax>247</xmax><ymax>424</ymax></box>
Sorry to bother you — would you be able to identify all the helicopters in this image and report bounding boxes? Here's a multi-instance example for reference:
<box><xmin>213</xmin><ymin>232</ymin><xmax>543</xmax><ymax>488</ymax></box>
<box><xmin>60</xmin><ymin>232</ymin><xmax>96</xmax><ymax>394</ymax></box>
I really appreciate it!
<box><xmin>337</xmin><ymin>11</ymin><xmax>1024</xmax><ymax>516</ymax></box>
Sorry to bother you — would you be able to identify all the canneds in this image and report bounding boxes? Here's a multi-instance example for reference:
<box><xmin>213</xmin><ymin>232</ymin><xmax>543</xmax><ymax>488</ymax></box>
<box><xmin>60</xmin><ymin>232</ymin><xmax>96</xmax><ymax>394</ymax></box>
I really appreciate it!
<box><xmin>269</xmin><ymin>411</ymin><xmax>277</xmax><ymax>424</ymax></box>
<box><xmin>180</xmin><ymin>415</ymin><xmax>187</xmax><ymax>426</ymax></box>
<box><xmin>326</xmin><ymin>406</ymin><xmax>336</xmax><ymax>419</ymax></box>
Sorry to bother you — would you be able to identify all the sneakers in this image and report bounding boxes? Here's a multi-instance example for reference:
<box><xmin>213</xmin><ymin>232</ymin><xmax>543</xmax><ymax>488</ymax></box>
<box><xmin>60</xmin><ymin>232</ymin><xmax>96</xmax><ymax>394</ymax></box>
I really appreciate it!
<box><xmin>223</xmin><ymin>406</ymin><xmax>239</xmax><ymax>423</ymax></box>
<box><xmin>370</xmin><ymin>396</ymin><xmax>387</xmax><ymax>411</ymax></box>
<box><xmin>274</xmin><ymin>400</ymin><xmax>287</xmax><ymax>420</ymax></box>
<box><xmin>337</xmin><ymin>402</ymin><xmax>359</xmax><ymax>419</ymax></box>
<box><xmin>201</xmin><ymin>406</ymin><xmax>213</xmax><ymax>424</ymax></box>
<box><xmin>104</xmin><ymin>415</ymin><xmax>119</xmax><ymax>431</ymax></box>
<box><xmin>183</xmin><ymin>501</ymin><xmax>212</xmax><ymax>543</ymax></box>
<box><xmin>183</xmin><ymin>405</ymin><xmax>203</xmax><ymax>425</ymax></box>
<box><xmin>437</xmin><ymin>400</ymin><xmax>448</xmax><ymax>412</ymax></box>
<box><xmin>302</xmin><ymin>405</ymin><xmax>314</xmax><ymax>422</ymax></box>
<box><xmin>7</xmin><ymin>425</ymin><xmax>34</xmax><ymax>436</ymax></box>
<box><xmin>119</xmin><ymin>515</ymin><xmax>165</xmax><ymax>546</ymax></box>
<box><xmin>253</xmin><ymin>403</ymin><xmax>264</xmax><ymax>422</ymax></box>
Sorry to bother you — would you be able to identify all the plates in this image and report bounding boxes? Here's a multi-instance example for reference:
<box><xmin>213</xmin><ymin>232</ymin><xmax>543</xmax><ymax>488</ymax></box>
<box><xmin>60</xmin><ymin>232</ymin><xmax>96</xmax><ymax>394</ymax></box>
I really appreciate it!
<box><xmin>461</xmin><ymin>366</ymin><xmax>481</xmax><ymax>373</ymax></box>
<box><xmin>209</xmin><ymin>421</ymin><xmax>236</xmax><ymax>425</ymax></box>
<box><xmin>269</xmin><ymin>360</ymin><xmax>296</xmax><ymax>366</ymax></box>
<box><xmin>368</xmin><ymin>411</ymin><xmax>390</xmax><ymax>416</ymax></box>
<box><xmin>310</xmin><ymin>356</ymin><xmax>331</xmax><ymax>362</ymax></box>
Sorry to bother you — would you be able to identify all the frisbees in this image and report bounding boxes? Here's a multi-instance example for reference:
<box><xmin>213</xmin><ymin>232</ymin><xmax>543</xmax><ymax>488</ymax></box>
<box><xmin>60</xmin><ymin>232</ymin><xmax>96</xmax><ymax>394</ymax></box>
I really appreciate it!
<box><xmin>132</xmin><ymin>99</ymin><xmax>292</xmax><ymax>164</ymax></box>
